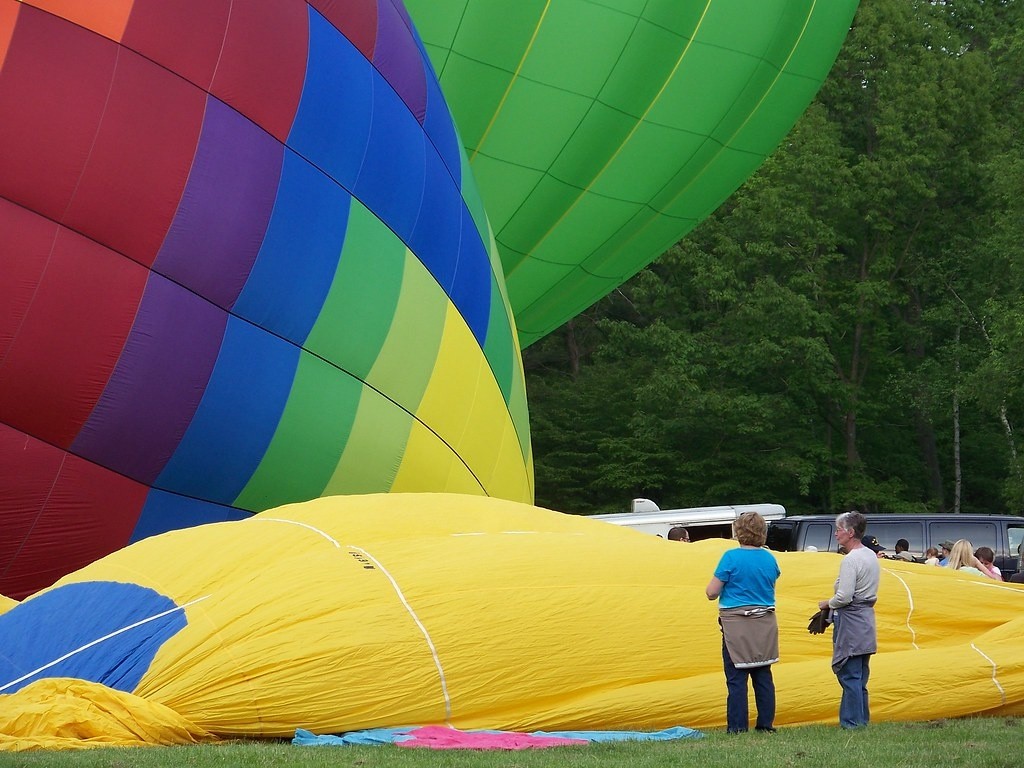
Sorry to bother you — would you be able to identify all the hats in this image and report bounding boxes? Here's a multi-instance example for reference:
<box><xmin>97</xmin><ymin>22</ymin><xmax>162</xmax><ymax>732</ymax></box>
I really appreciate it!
<box><xmin>860</xmin><ymin>535</ymin><xmax>886</xmax><ymax>551</ymax></box>
<box><xmin>938</xmin><ymin>539</ymin><xmax>955</xmax><ymax>551</ymax></box>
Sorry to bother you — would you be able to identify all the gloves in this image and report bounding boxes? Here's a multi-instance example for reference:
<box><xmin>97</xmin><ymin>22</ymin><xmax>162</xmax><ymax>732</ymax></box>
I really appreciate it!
<box><xmin>807</xmin><ymin>608</ymin><xmax>831</xmax><ymax>635</ymax></box>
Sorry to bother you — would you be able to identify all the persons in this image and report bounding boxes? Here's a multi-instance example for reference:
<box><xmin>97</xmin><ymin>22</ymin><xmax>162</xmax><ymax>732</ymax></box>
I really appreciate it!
<box><xmin>862</xmin><ymin>535</ymin><xmax>1004</xmax><ymax>581</ymax></box>
<box><xmin>818</xmin><ymin>511</ymin><xmax>880</xmax><ymax>730</ymax></box>
<box><xmin>667</xmin><ymin>527</ymin><xmax>691</xmax><ymax>543</ymax></box>
<box><xmin>705</xmin><ymin>511</ymin><xmax>781</xmax><ymax>735</ymax></box>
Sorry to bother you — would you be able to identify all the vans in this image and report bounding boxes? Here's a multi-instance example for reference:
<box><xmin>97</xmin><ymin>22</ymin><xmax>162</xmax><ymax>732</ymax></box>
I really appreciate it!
<box><xmin>764</xmin><ymin>510</ymin><xmax>1024</xmax><ymax>584</ymax></box>
<box><xmin>581</xmin><ymin>496</ymin><xmax>786</xmax><ymax>543</ymax></box>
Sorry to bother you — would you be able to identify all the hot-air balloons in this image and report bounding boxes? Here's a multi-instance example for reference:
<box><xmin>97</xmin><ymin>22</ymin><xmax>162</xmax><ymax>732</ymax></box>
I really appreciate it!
<box><xmin>400</xmin><ymin>0</ymin><xmax>858</xmax><ymax>354</ymax></box>
<box><xmin>0</xmin><ymin>1</ymin><xmax>538</xmax><ymax>611</ymax></box>
<box><xmin>0</xmin><ymin>493</ymin><xmax>1024</xmax><ymax>753</ymax></box>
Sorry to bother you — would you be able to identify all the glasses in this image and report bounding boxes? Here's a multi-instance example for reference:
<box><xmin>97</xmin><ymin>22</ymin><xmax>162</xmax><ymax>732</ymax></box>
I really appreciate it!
<box><xmin>836</xmin><ymin>527</ymin><xmax>847</xmax><ymax>533</ymax></box>
